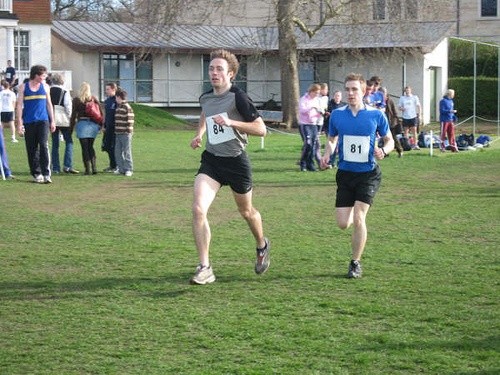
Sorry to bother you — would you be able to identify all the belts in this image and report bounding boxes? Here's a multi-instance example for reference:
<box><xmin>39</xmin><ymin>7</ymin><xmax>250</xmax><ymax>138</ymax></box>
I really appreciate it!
<box><xmin>78</xmin><ymin>119</ymin><xmax>93</xmax><ymax>121</ymax></box>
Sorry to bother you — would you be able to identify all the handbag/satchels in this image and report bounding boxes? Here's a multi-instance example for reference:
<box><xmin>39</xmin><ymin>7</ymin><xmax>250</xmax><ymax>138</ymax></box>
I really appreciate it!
<box><xmin>53</xmin><ymin>89</ymin><xmax>71</xmax><ymax>128</ymax></box>
<box><xmin>86</xmin><ymin>97</ymin><xmax>103</xmax><ymax>124</ymax></box>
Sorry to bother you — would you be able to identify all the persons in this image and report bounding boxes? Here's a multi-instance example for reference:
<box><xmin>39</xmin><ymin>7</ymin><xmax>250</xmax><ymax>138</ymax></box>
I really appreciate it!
<box><xmin>101</xmin><ymin>82</ymin><xmax>119</xmax><ymax>173</ymax></box>
<box><xmin>0</xmin><ymin>60</ymin><xmax>18</xmax><ymax>142</ymax></box>
<box><xmin>17</xmin><ymin>65</ymin><xmax>55</xmax><ymax>184</ymax></box>
<box><xmin>321</xmin><ymin>73</ymin><xmax>394</xmax><ymax>279</ymax></box>
<box><xmin>49</xmin><ymin>74</ymin><xmax>79</xmax><ymax>174</ymax></box>
<box><xmin>114</xmin><ymin>87</ymin><xmax>134</xmax><ymax>177</ymax></box>
<box><xmin>439</xmin><ymin>89</ymin><xmax>459</xmax><ymax>153</ymax></box>
<box><xmin>191</xmin><ymin>50</ymin><xmax>270</xmax><ymax>284</ymax></box>
<box><xmin>69</xmin><ymin>82</ymin><xmax>105</xmax><ymax>174</ymax></box>
<box><xmin>297</xmin><ymin>81</ymin><xmax>421</xmax><ymax>172</ymax></box>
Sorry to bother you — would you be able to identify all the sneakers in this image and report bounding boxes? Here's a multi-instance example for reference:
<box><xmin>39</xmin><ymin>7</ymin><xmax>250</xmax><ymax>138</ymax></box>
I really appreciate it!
<box><xmin>348</xmin><ymin>260</ymin><xmax>361</xmax><ymax>278</ymax></box>
<box><xmin>44</xmin><ymin>176</ymin><xmax>51</xmax><ymax>183</ymax></box>
<box><xmin>190</xmin><ymin>264</ymin><xmax>216</xmax><ymax>285</ymax></box>
<box><xmin>255</xmin><ymin>237</ymin><xmax>269</xmax><ymax>274</ymax></box>
<box><xmin>33</xmin><ymin>174</ymin><xmax>44</xmax><ymax>183</ymax></box>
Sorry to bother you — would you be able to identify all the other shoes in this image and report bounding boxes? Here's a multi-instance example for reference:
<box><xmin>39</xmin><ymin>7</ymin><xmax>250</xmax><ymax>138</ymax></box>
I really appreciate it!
<box><xmin>103</xmin><ymin>167</ymin><xmax>118</xmax><ymax>173</ymax></box>
<box><xmin>12</xmin><ymin>138</ymin><xmax>19</xmax><ymax>142</ymax></box>
<box><xmin>7</xmin><ymin>175</ymin><xmax>16</xmax><ymax>180</ymax></box>
<box><xmin>68</xmin><ymin>168</ymin><xmax>79</xmax><ymax>173</ymax></box>
<box><xmin>125</xmin><ymin>171</ymin><xmax>133</xmax><ymax>177</ymax></box>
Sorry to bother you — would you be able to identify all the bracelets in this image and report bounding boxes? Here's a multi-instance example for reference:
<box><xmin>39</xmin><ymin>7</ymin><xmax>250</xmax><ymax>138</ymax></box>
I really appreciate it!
<box><xmin>382</xmin><ymin>148</ymin><xmax>387</xmax><ymax>156</ymax></box>
